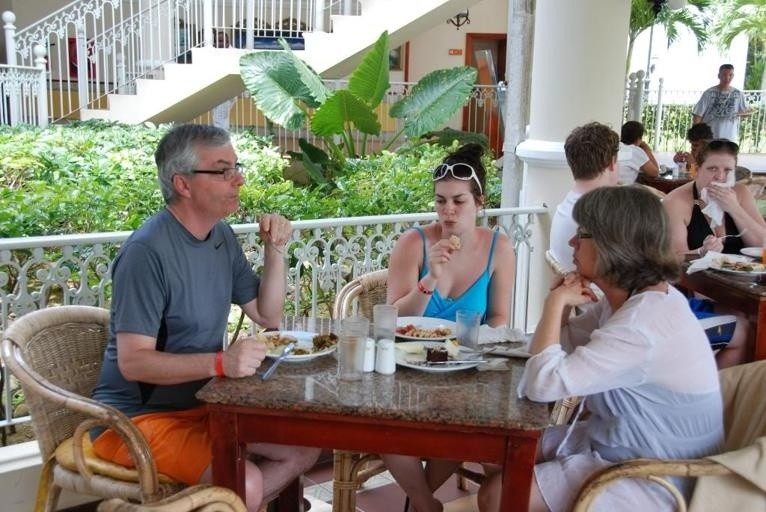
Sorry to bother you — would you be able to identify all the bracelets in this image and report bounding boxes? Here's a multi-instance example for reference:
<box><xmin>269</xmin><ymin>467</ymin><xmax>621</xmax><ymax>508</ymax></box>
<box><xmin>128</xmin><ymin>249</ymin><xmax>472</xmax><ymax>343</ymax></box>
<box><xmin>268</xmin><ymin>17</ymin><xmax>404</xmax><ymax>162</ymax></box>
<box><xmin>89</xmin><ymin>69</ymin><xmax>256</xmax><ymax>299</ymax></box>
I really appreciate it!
<box><xmin>416</xmin><ymin>281</ymin><xmax>435</xmax><ymax>297</ymax></box>
<box><xmin>213</xmin><ymin>351</ymin><xmax>228</xmax><ymax>379</ymax></box>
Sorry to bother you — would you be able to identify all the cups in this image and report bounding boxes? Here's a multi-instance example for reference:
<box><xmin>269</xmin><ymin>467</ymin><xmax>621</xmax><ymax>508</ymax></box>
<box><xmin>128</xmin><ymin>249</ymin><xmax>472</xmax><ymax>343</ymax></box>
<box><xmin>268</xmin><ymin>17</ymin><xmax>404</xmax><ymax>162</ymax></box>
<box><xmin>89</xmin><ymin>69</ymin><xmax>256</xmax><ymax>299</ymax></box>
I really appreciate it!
<box><xmin>456</xmin><ymin>310</ymin><xmax>481</xmax><ymax>354</ymax></box>
<box><xmin>677</xmin><ymin>163</ymin><xmax>687</xmax><ymax>178</ymax></box>
<box><xmin>372</xmin><ymin>304</ymin><xmax>397</xmax><ymax>343</ymax></box>
<box><xmin>338</xmin><ymin>316</ymin><xmax>365</xmax><ymax>380</ymax></box>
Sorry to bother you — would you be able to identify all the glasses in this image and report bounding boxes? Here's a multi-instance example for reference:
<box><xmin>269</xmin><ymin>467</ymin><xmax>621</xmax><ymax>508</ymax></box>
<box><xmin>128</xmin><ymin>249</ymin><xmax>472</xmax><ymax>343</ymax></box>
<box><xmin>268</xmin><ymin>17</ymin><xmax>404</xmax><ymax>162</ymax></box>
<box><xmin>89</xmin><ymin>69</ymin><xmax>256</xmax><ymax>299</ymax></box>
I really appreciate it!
<box><xmin>575</xmin><ymin>231</ymin><xmax>592</xmax><ymax>245</ymax></box>
<box><xmin>193</xmin><ymin>162</ymin><xmax>245</xmax><ymax>181</ymax></box>
<box><xmin>432</xmin><ymin>161</ymin><xmax>482</xmax><ymax>194</ymax></box>
<box><xmin>704</xmin><ymin>140</ymin><xmax>738</xmax><ymax>153</ymax></box>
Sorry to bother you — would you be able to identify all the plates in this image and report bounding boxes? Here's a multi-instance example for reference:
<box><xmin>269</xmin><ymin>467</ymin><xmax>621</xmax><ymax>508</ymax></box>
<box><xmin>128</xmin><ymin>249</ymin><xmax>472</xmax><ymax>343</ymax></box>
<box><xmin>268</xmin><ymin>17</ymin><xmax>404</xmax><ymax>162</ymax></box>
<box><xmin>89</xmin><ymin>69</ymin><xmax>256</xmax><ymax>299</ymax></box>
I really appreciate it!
<box><xmin>707</xmin><ymin>247</ymin><xmax>766</xmax><ymax>276</ymax></box>
<box><xmin>390</xmin><ymin>313</ymin><xmax>466</xmax><ymax>339</ymax></box>
<box><xmin>256</xmin><ymin>329</ymin><xmax>337</xmax><ymax>362</ymax></box>
<box><xmin>734</xmin><ymin>109</ymin><xmax>759</xmax><ymax>116</ymax></box>
<box><xmin>392</xmin><ymin>341</ymin><xmax>484</xmax><ymax>372</ymax></box>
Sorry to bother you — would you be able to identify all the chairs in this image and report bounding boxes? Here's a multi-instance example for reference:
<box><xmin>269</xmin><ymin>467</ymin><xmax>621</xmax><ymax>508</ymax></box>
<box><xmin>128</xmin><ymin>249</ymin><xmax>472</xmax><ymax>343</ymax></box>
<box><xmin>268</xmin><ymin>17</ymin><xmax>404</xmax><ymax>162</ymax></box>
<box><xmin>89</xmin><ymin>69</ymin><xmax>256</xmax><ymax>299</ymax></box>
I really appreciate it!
<box><xmin>0</xmin><ymin>304</ymin><xmax>186</xmax><ymax>512</ymax></box>
<box><xmin>93</xmin><ymin>483</ymin><xmax>250</xmax><ymax>512</ymax></box>
<box><xmin>330</xmin><ymin>267</ymin><xmax>486</xmax><ymax>512</ymax></box>
<box><xmin>736</xmin><ymin>175</ymin><xmax>766</xmax><ymax>203</ymax></box>
<box><xmin>568</xmin><ymin>361</ymin><xmax>765</xmax><ymax>512</ymax></box>
<box><xmin>734</xmin><ymin>165</ymin><xmax>751</xmax><ymax>185</ymax></box>
<box><xmin>543</xmin><ymin>249</ymin><xmax>569</xmax><ymax>282</ymax></box>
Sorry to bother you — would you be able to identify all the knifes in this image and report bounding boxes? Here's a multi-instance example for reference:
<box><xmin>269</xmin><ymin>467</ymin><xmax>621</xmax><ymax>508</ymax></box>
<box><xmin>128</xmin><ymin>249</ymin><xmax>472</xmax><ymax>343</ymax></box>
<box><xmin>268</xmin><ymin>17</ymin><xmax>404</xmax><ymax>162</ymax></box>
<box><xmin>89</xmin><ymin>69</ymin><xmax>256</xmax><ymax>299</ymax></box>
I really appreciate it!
<box><xmin>261</xmin><ymin>341</ymin><xmax>293</xmax><ymax>381</ymax></box>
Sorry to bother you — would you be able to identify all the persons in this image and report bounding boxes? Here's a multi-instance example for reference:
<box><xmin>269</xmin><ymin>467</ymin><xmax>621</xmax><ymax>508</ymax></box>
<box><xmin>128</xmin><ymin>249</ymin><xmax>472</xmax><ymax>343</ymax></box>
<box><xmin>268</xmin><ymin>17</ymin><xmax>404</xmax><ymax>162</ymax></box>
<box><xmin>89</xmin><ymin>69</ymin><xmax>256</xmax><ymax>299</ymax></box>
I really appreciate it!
<box><xmin>614</xmin><ymin>121</ymin><xmax>663</xmax><ymax>187</ymax></box>
<box><xmin>664</xmin><ymin>137</ymin><xmax>763</xmax><ymax>265</ymax></box>
<box><xmin>87</xmin><ymin>121</ymin><xmax>325</xmax><ymax>511</ymax></box>
<box><xmin>673</xmin><ymin>123</ymin><xmax>715</xmax><ymax>177</ymax></box>
<box><xmin>373</xmin><ymin>141</ymin><xmax>517</xmax><ymax>512</ymax></box>
<box><xmin>548</xmin><ymin>119</ymin><xmax>753</xmax><ymax>369</ymax></box>
<box><xmin>693</xmin><ymin>63</ymin><xmax>752</xmax><ymax>147</ymax></box>
<box><xmin>476</xmin><ymin>184</ymin><xmax>728</xmax><ymax>512</ymax></box>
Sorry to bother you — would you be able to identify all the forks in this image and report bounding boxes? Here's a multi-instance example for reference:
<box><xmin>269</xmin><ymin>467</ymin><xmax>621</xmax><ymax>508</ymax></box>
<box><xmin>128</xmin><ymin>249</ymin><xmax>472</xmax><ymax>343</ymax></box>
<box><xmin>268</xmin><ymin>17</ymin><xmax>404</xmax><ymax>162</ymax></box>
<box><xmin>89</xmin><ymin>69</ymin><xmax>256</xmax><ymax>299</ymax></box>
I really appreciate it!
<box><xmin>715</xmin><ymin>229</ymin><xmax>750</xmax><ymax>240</ymax></box>
<box><xmin>404</xmin><ymin>357</ymin><xmax>488</xmax><ymax>366</ymax></box>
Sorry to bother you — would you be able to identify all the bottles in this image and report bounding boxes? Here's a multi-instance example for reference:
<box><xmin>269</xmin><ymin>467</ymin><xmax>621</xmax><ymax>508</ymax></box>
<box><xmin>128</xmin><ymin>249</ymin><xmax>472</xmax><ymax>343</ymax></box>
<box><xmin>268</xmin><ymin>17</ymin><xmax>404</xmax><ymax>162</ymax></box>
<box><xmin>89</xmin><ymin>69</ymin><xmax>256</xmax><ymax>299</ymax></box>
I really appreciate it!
<box><xmin>356</xmin><ymin>338</ymin><xmax>397</xmax><ymax>375</ymax></box>
